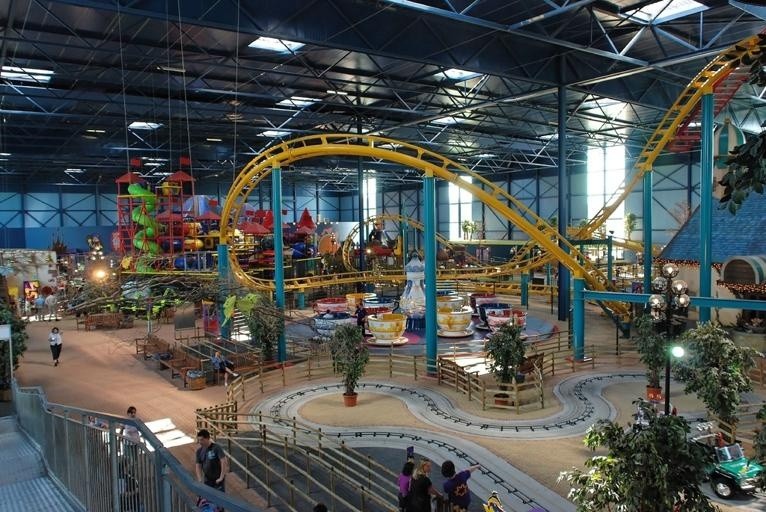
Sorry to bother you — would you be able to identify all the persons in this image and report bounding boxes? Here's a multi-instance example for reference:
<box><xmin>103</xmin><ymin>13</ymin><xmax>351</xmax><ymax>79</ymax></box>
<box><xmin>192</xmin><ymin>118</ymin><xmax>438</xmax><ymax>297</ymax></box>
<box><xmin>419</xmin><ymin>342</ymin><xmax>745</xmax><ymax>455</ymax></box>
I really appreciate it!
<box><xmin>406</xmin><ymin>459</ymin><xmax>443</xmax><ymax>512</ymax></box>
<box><xmin>87</xmin><ymin>414</ymin><xmax>108</xmax><ymax>450</ymax></box>
<box><xmin>45</xmin><ymin>292</ymin><xmax>59</xmax><ymax>322</ymax></box>
<box><xmin>212</xmin><ymin>351</ymin><xmax>239</xmax><ymax>386</ymax></box>
<box><xmin>322</xmin><ymin>309</ymin><xmax>334</xmax><ymax>319</ymax></box>
<box><xmin>110</xmin><ymin>456</ymin><xmax>145</xmax><ymax>512</ymax></box>
<box><xmin>47</xmin><ymin>326</ymin><xmax>63</xmax><ymax>366</ymax></box>
<box><xmin>194</xmin><ymin>428</ymin><xmax>227</xmax><ymax>492</ymax></box>
<box><xmin>24</xmin><ymin>296</ymin><xmax>32</xmax><ymax>320</ymax></box>
<box><xmin>35</xmin><ymin>294</ymin><xmax>45</xmax><ymax>321</ymax></box>
<box><xmin>196</xmin><ymin>494</ymin><xmax>227</xmax><ymax>512</ymax></box>
<box><xmin>352</xmin><ymin>302</ymin><xmax>367</xmax><ymax>337</ymax></box>
<box><xmin>398</xmin><ymin>461</ymin><xmax>415</xmax><ymax>497</ymax></box>
<box><xmin>117</xmin><ymin>405</ymin><xmax>141</xmax><ymax>478</ymax></box>
<box><xmin>442</xmin><ymin>460</ymin><xmax>481</xmax><ymax>509</ymax></box>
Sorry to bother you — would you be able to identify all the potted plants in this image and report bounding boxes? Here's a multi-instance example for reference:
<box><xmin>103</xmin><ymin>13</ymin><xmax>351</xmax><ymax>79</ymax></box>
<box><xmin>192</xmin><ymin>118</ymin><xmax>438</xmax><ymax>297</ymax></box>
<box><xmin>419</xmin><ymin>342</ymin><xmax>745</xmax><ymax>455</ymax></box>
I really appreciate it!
<box><xmin>628</xmin><ymin>310</ymin><xmax>685</xmax><ymax>404</ymax></box>
<box><xmin>329</xmin><ymin>320</ymin><xmax>370</xmax><ymax>409</ymax></box>
<box><xmin>482</xmin><ymin>321</ymin><xmax>527</xmax><ymax>408</ymax></box>
<box><xmin>0</xmin><ymin>289</ymin><xmax>28</xmax><ymax>406</ymax></box>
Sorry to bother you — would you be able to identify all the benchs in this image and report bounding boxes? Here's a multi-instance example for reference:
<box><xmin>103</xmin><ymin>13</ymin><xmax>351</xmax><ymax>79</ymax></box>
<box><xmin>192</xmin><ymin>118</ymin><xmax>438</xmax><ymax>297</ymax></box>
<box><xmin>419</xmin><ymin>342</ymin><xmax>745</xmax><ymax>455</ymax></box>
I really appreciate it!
<box><xmin>133</xmin><ymin>336</ymin><xmax>171</xmax><ymax>365</ymax></box>
<box><xmin>209</xmin><ymin>350</ymin><xmax>259</xmax><ymax>387</ymax></box>
<box><xmin>78</xmin><ymin>311</ymin><xmax>125</xmax><ymax>332</ymax></box>
<box><xmin>163</xmin><ymin>348</ymin><xmax>187</xmax><ymax>380</ymax></box>
<box><xmin>174</xmin><ymin>355</ymin><xmax>207</xmax><ymax>388</ymax></box>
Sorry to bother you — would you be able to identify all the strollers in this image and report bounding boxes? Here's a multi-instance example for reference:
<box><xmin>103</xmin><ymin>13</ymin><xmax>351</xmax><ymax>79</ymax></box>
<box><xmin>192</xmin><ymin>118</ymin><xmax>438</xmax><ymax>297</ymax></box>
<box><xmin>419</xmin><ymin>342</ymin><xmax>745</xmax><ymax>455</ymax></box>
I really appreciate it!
<box><xmin>194</xmin><ymin>480</ymin><xmax>226</xmax><ymax>512</ymax></box>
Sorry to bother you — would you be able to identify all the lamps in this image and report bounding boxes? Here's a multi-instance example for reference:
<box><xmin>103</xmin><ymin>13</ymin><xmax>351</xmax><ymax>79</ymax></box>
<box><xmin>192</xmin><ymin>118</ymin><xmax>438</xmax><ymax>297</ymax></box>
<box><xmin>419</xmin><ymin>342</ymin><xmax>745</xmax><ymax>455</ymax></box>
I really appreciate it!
<box><xmin>712</xmin><ymin>89</ymin><xmax>746</xmax><ymax>159</ymax></box>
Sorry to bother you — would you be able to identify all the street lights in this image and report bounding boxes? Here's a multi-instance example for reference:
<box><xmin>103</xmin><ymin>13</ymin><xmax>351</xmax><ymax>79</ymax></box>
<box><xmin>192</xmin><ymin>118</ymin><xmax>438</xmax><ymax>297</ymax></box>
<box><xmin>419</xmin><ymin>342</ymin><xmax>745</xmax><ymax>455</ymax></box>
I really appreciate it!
<box><xmin>648</xmin><ymin>262</ymin><xmax>691</xmax><ymax>420</ymax></box>
